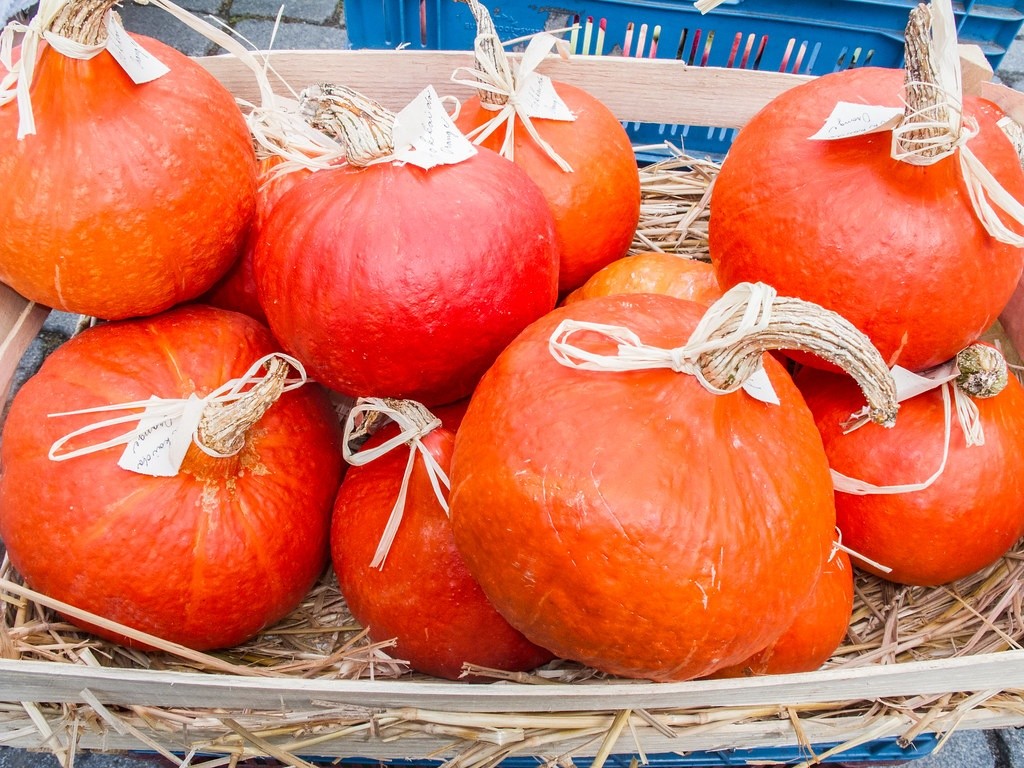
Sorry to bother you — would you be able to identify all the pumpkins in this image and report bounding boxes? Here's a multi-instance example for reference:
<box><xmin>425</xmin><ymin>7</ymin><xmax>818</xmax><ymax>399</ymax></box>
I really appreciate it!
<box><xmin>0</xmin><ymin>0</ymin><xmax>1023</xmax><ymax>677</ymax></box>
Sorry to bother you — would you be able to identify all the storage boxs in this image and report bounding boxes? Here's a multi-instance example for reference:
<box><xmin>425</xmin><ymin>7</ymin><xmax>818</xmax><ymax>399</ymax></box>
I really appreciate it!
<box><xmin>342</xmin><ymin>0</ymin><xmax>1023</xmax><ymax>183</ymax></box>
<box><xmin>1</xmin><ymin>42</ymin><xmax>1024</xmax><ymax>730</ymax></box>
